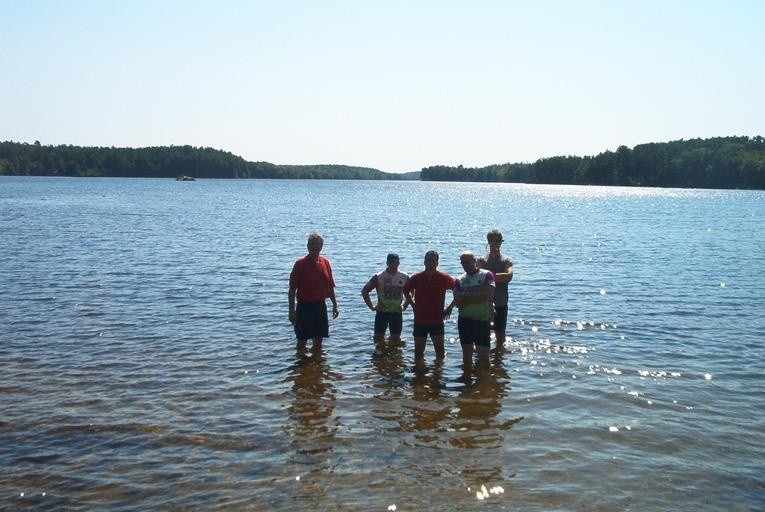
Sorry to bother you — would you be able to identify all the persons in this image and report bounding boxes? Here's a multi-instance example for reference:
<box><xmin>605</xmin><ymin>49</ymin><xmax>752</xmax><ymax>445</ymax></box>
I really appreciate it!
<box><xmin>403</xmin><ymin>250</ymin><xmax>457</xmax><ymax>359</ymax></box>
<box><xmin>287</xmin><ymin>233</ymin><xmax>338</xmax><ymax>354</ymax></box>
<box><xmin>452</xmin><ymin>250</ymin><xmax>496</xmax><ymax>364</ymax></box>
<box><xmin>361</xmin><ymin>254</ymin><xmax>413</xmax><ymax>341</ymax></box>
<box><xmin>475</xmin><ymin>229</ymin><xmax>513</xmax><ymax>345</ymax></box>
